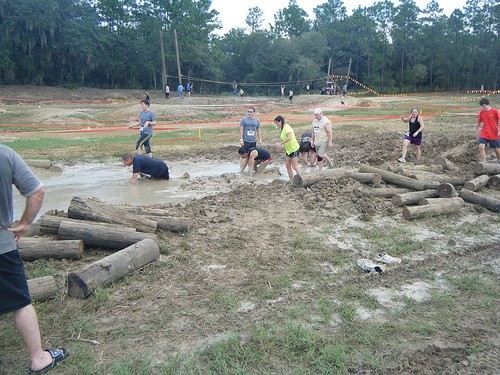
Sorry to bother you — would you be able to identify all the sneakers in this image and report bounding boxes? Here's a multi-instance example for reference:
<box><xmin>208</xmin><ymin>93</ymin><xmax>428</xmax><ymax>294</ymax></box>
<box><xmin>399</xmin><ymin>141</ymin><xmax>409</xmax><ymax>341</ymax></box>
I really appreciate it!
<box><xmin>397</xmin><ymin>156</ymin><xmax>407</xmax><ymax>163</ymax></box>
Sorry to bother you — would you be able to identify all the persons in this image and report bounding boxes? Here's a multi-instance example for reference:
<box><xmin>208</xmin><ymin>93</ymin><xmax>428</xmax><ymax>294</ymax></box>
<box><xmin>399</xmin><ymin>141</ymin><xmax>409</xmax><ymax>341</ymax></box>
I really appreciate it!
<box><xmin>128</xmin><ymin>100</ymin><xmax>158</xmax><ymax>157</ymax></box>
<box><xmin>297</xmin><ymin>131</ymin><xmax>322</xmax><ymax>172</ymax></box>
<box><xmin>232</xmin><ymin>81</ymin><xmax>237</xmax><ymax>96</ymax></box>
<box><xmin>330</xmin><ymin>83</ymin><xmax>349</xmax><ymax>108</ymax></box>
<box><xmin>165</xmin><ymin>84</ymin><xmax>170</xmax><ymax>100</ymax></box>
<box><xmin>145</xmin><ymin>90</ymin><xmax>151</xmax><ymax>106</ymax></box>
<box><xmin>240</xmin><ymin>88</ymin><xmax>244</xmax><ymax>97</ymax></box>
<box><xmin>122</xmin><ymin>153</ymin><xmax>170</xmax><ymax>184</ymax></box>
<box><xmin>0</xmin><ymin>144</ymin><xmax>71</xmax><ymax>374</ymax></box>
<box><xmin>476</xmin><ymin>98</ymin><xmax>500</xmax><ymax>166</ymax></box>
<box><xmin>177</xmin><ymin>82</ymin><xmax>185</xmax><ymax>101</ymax></box>
<box><xmin>240</xmin><ymin>105</ymin><xmax>263</xmax><ymax>148</ymax></box>
<box><xmin>306</xmin><ymin>84</ymin><xmax>310</xmax><ymax>94</ymax></box>
<box><xmin>281</xmin><ymin>85</ymin><xmax>286</xmax><ymax>100</ymax></box>
<box><xmin>238</xmin><ymin>147</ymin><xmax>282</xmax><ymax>179</ymax></box>
<box><xmin>311</xmin><ymin>107</ymin><xmax>336</xmax><ymax>170</ymax></box>
<box><xmin>396</xmin><ymin>107</ymin><xmax>425</xmax><ymax>163</ymax></box>
<box><xmin>288</xmin><ymin>90</ymin><xmax>293</xmax><ymax>104</ymax></box>
<box><xmin>274</xmin><ymin>115</ymin><xmax>301</xmax><ymax>184</ymax></box>
<box><xmin>186</xmin><ymin>82</ymin><xmax>193</xmax><ymax>98</ymax></box>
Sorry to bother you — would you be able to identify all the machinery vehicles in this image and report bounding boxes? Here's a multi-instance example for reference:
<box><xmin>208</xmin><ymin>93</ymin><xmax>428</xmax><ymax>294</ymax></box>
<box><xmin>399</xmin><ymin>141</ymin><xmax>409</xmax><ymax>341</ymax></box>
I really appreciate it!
<box><xmin>321</xmin><ymin>82</ymin><xmax>336</xmax><ymax>95</ymax></box>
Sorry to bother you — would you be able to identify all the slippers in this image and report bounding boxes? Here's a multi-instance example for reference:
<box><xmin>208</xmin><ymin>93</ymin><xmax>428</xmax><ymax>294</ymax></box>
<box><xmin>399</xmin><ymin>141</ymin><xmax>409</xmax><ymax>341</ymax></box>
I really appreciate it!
<box><xmin>29</xmin><ymin>347</ymin><xmax>69</xmax><ymax>374</ymax></box>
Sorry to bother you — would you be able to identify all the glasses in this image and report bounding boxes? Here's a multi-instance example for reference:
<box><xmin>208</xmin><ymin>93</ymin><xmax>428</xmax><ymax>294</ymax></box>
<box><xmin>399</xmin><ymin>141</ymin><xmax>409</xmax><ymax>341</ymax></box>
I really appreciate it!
<box><xmin>247</xmin><ymin>110</ymin><xmax>252</xmax><ymax>113</ymax></box>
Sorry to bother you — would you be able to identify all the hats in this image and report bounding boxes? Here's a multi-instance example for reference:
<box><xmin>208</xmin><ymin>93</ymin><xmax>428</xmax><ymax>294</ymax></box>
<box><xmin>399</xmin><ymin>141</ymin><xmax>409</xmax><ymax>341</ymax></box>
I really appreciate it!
<box><xmin>314</xmin><ymin>107</ymin><xmax>322</xmax><ymax>114</ymax></box>
